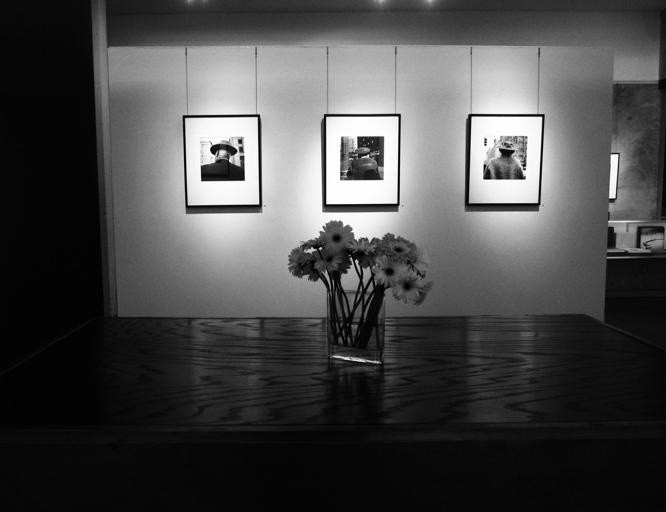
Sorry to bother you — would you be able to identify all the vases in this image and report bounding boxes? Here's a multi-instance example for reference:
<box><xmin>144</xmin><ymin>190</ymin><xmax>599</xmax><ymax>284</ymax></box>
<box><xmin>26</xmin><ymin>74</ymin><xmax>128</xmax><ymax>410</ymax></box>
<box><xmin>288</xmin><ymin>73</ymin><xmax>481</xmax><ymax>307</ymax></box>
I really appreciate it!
<box><xmin>326</xmin><ymin>291</ymin><xmax>385</xmax><ymax>371</ymax></box>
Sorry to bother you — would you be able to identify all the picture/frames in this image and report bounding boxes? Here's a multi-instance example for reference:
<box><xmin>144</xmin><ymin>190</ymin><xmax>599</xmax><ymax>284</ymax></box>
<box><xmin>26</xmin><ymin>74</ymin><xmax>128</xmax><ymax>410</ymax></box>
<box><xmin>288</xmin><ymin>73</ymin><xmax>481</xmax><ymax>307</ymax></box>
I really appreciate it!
<box><xmin>183</xmin><ymin>114</ymin><xmax>261</xmax><ymax>208</ymax></box>
<box><xmin>324</xmin><ymin>114</ymin><xmax>401</xmax><ymax>206</ymax></box>
<box><xmin>465</xmin><ymin>114</ymin><xmax>545</xmax><ymax>206</ymax></box>
<box><xmin>609</xmin><ymin>152</ymin><xmax>620</xmax><ymax>200</ymax></box>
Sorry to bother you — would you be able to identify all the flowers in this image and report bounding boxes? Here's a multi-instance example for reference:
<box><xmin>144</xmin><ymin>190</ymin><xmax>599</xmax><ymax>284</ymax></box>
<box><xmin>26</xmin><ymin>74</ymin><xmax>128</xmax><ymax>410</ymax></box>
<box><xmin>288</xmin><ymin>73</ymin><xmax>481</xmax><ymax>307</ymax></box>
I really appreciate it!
<box><xmin>288</xmin><ymin>221</ymin><xmax>433</xmax><ymax>350</ymax></box>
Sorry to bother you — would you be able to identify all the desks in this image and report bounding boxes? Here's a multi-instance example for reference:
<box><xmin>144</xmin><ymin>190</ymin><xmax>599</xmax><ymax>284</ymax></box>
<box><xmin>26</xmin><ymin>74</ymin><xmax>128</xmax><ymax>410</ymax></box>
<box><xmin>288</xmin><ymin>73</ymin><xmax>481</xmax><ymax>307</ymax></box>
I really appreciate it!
<box><xmin>1</xmin><ymin>314</ymin><xmax>666</xmax><ymax>511</ymax></box>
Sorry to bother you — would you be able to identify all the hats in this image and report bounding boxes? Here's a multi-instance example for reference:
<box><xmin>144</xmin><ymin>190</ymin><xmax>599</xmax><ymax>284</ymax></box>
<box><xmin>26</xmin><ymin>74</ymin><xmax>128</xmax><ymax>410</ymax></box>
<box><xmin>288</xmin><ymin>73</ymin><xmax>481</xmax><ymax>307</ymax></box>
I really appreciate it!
<box><xmin>499</xmin><ymin>141</ymin><xmax>516</xmax><ymax>150</ymax></box>
<box><xmin>356</xmin><ymin>147</ymin><xmax>370</xmax><ymax>154</ymax></box>
<box><xmin>210</xmin><ymin>140</ymin><xmax>237</xmax><ymax>155</ymax></box>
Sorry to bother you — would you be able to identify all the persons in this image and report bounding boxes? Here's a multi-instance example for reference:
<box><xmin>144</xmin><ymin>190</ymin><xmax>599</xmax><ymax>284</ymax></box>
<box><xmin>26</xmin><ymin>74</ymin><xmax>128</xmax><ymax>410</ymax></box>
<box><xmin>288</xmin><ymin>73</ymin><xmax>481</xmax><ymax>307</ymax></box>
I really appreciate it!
<box><xmin>200</xmin><ymin>139</ymin><xmax>246</xmax><ymax>181</ymax></box>
<box><xmin>346</xmin><ymin>146</ymin><xmax>379</xmax><ymax>179</ymax></box>
<box><xmin>483</xmin><ymin>142</ymin><xmax>525</xmax><ymax>179</ymax></box>
